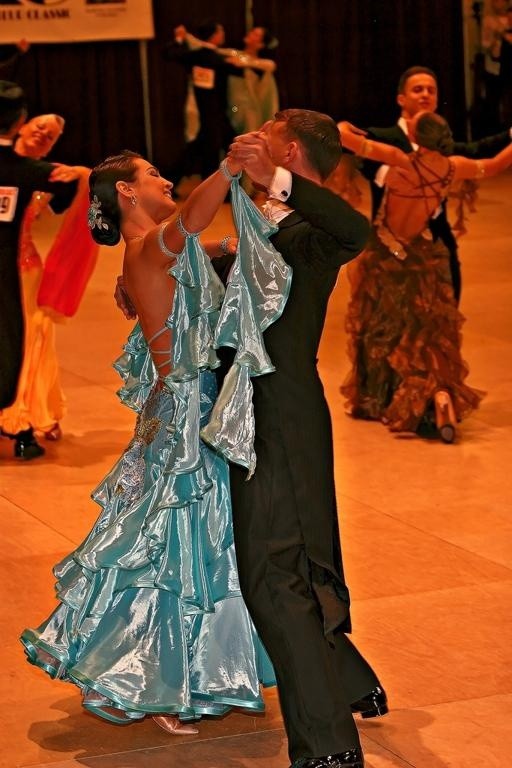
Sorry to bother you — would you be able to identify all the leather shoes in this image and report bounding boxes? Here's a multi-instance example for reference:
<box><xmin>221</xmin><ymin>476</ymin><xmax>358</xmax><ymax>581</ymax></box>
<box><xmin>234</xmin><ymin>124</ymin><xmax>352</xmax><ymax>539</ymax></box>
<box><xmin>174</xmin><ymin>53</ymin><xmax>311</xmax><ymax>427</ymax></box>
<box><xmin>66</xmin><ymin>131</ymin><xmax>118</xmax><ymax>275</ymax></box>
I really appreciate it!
<box><xmin>16</xmin><ymin>426</ymin><xmax>60</xmax><ymax>458</ymax></box>
<box><xmin>152</xmin><ymin>714</ymin><xmax>199</xmax><ymax>735</ymax></box>
<box><xmin>289</xmin><ymin>747</ymin><xmax>364</xmax><ymax>768</ymax></box>
<box><xmin>351</xmin><ymin>686</ymin><xmax>387</xmax><ymax>718</ymax></box>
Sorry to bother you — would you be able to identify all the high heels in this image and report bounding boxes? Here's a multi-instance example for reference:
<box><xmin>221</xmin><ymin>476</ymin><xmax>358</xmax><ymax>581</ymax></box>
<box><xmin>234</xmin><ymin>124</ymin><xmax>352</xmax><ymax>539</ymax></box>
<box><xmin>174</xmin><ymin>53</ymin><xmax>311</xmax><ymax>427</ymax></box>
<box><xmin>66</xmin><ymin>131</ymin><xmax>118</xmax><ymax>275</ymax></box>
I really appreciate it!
<box><xmin>435</xmin><ymin>391</ymin><xmax>457</xmax><ymax>443</ymax></box>
